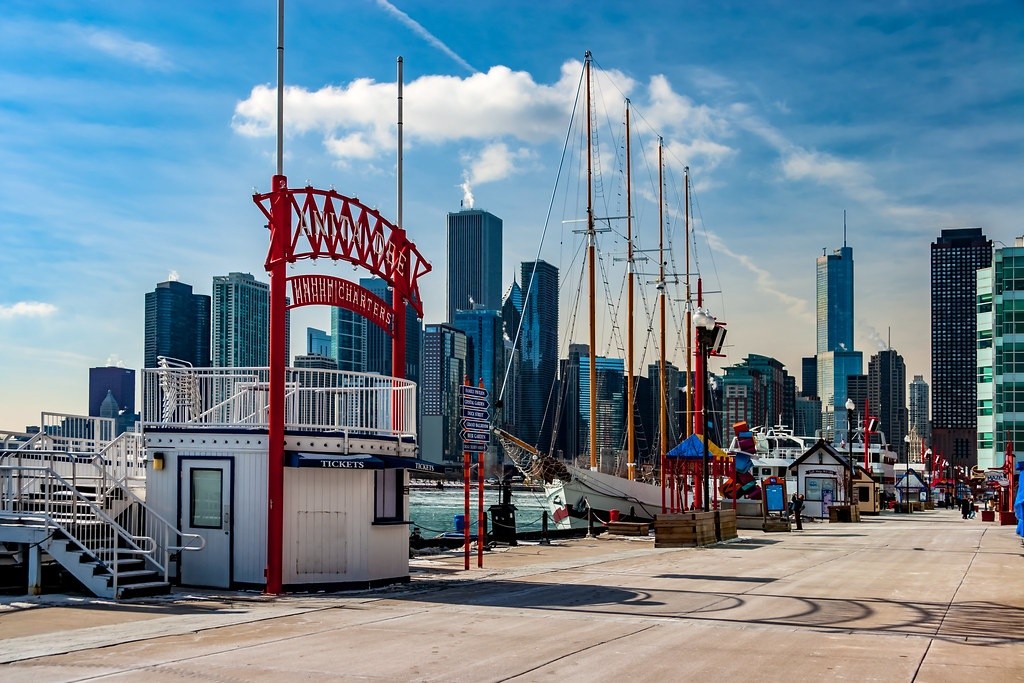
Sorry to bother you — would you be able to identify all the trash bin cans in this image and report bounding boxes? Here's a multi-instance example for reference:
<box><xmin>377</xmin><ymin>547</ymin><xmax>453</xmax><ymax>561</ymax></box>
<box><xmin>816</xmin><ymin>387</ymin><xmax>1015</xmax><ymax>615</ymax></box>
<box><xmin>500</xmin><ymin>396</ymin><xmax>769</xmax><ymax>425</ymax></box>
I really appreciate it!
<box><xmin>835</xmin><ymin>510</ymin><xmax>848</xmax><ymax>522</ymax></box>
<box><xmin>893</xmin><ymin>504</ymin><xmax>900</xmax><ymax>513</ymax></box>
<box><xmin>488</xmin><ymin>504</ymin><xmax>519</xmax><ymax>545</ymax></box>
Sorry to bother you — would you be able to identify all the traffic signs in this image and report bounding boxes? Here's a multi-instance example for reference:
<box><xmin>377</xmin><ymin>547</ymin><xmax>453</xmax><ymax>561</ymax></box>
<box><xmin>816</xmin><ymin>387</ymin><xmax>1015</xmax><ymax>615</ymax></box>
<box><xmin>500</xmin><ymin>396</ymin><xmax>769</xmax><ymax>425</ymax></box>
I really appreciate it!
<box><xmin>456</xmin><ymin>385</ymin><xmax>492</xmax><ymax>454</ymax></box>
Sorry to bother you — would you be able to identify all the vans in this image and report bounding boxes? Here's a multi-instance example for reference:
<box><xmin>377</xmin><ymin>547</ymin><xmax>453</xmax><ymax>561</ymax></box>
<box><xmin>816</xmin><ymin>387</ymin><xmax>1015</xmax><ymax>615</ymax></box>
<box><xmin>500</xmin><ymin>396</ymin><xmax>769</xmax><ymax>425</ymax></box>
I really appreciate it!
<box><xmin>511</xmin><ymin>476</ymin><xmax>523</xmax><ymax>480</ymax></box>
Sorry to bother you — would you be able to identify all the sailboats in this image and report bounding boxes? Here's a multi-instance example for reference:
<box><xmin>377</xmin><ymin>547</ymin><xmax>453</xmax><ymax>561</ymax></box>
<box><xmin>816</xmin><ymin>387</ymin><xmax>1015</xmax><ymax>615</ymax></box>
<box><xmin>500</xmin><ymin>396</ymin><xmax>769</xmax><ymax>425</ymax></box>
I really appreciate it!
<box><xmin>492</xmin><ymin>52</ymin><xmax>731</xmax><ymax>530</ymax></box>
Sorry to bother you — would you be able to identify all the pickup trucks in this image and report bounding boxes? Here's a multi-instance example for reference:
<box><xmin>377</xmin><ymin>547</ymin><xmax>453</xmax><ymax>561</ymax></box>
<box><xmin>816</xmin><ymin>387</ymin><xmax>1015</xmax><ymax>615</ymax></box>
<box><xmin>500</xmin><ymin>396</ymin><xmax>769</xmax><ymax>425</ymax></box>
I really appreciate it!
<box><xmin>484</xmin><ymin>478</ymin><xmax>497</xmax><ymax>483</ymax></box>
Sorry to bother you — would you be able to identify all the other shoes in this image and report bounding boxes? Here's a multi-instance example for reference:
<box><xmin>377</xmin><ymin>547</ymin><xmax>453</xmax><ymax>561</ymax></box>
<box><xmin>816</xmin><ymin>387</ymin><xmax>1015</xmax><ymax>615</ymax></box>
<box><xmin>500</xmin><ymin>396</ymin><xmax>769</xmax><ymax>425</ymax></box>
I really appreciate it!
<box><xmin>795</xmin><ymin>529</ymin><xmax>802</xmax><ymax>530</ymax></box>
<box><xmin>968</xmin><ymin>516</ymin><xmax>970</xmax><ymax>518</ymax></box>
<box><xmin>962</xmin><ymin>517</ymin><xmax>964</xmax><ymax>519</ymax></box>
<box><xmin>965</xmin><ymin>518</ymin><xmax>967</xmax><ymax>519</ymax></box>
<box><xmin>970</xmin><ymin>517</ymin><xmax>973</xmax><ymax>519</ymax></box>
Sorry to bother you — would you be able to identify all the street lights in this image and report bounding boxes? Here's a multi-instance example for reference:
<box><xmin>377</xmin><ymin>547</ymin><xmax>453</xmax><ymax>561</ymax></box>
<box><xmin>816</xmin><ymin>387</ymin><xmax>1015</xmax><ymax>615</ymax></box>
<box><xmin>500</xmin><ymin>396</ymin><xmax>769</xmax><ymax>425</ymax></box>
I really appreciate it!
<box><xmin>844</xmin><ymin>398</ymin><xmax>855</xmax><ymax>505</ymax></box>
<box><xmin>945</xmin><ymin>461</ymin><xmax>949</xmax><ymax>502</ymax></box>
<box><xmin>955</xmin><ymin>466</ymin><xmax>958</xmax><ymax>501</ymax></box>
<box><xmin>960</xmin><ymin>469</ymin><xmax>964</xmax><ymax>500</ymax></box>
<box><xmin>927</xmin><ymin>449</ymin><xmax>933</xmax><ymax>501</ymax></box>
<box><xmin>690</xmin><ymin>308</ymin><xmax>715</xmax><ymax>510</ymax></box>
<box><xmin>903</xmin><ymin>436</ymin><xmax>911</xmax><ymax>503</ymax></box>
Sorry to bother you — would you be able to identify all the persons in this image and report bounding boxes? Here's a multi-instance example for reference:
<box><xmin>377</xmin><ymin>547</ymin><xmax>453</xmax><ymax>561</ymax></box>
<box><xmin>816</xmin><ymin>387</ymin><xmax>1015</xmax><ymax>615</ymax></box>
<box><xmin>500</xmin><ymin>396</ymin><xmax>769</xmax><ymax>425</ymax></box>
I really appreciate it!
<box><xmin>962</xmin><ymin>495</ymin><xmax>975</xmax><ymax>519</ymax></box>
<box><xmin>792</xmin><ymin>492</ymin><xmax>804</xmax><ymax>531</ymax></box>
<box><xmin>944</xmin><ymin>495</ymin><xmax>961</xmax><ymax>511</ymax></box>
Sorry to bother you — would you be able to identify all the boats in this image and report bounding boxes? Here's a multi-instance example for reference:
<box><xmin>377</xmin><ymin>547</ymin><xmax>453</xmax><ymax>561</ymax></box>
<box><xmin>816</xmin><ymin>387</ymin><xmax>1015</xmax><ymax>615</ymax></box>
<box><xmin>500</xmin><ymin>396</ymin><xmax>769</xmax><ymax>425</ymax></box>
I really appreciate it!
<box><xmin>688</xmin><ymin>414</ymin><xmax>926</xmax><ymax>512</ymax></box>
